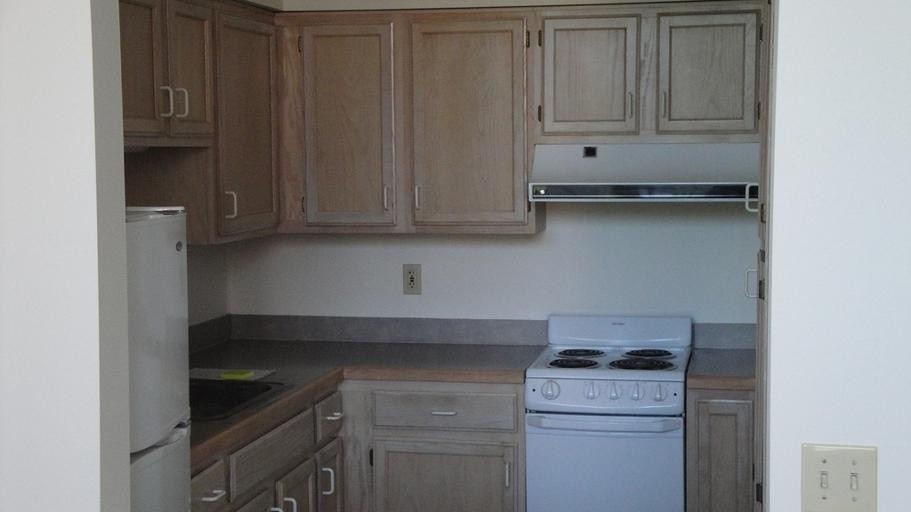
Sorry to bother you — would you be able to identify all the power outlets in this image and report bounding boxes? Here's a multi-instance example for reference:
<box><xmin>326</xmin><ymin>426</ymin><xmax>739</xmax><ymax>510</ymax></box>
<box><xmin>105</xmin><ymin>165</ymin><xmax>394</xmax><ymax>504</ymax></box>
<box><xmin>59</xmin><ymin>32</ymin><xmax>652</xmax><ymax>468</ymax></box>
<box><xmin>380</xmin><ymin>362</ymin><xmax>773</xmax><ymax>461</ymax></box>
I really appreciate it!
<box><xmin>403</xmin><ymin>264</ymin><xmax>422</xmax><ymax>295</ymax></box>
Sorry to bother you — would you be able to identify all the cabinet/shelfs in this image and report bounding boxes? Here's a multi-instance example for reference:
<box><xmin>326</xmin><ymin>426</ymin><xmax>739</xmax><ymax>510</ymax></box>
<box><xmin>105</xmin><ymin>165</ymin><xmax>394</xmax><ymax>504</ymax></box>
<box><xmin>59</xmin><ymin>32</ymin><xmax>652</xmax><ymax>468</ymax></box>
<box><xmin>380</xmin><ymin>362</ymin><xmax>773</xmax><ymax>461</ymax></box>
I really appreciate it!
<box><xmin>685</xmin><ymin>389</ymin><xmax>756</xmax><ymax>512</ymax></box>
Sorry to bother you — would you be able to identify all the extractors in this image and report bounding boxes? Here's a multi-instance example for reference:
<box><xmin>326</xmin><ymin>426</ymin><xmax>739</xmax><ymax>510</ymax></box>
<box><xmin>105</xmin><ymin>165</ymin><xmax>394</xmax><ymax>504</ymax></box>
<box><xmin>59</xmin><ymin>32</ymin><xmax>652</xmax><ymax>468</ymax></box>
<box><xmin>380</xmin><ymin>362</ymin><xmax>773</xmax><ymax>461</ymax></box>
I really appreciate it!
<box><xmin>523</xmin><ymin>141</ymin><xmax>763</xmax><ymax>206</ymax></box>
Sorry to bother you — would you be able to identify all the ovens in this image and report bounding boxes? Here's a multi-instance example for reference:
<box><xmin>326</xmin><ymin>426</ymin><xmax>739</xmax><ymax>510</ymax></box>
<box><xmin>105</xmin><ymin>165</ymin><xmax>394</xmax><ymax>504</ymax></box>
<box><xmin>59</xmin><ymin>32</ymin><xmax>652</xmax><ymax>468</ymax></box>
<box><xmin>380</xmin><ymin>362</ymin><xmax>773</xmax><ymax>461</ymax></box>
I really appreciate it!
<box><xmin>524</xmin><ymin>414</ymin><xmax>687</xmax><ymax>510</ymax></box>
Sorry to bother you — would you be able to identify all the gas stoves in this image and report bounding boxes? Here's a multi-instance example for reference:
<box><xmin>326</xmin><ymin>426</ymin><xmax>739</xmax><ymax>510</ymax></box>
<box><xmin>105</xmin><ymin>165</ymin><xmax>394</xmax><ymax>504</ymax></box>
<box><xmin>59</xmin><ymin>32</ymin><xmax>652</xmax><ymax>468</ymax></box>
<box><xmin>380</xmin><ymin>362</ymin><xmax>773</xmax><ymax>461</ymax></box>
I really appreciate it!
<box><xmin>524</xmin><ymin>347</ymin><xmax>693</xmax><ymax>382</ymax></box>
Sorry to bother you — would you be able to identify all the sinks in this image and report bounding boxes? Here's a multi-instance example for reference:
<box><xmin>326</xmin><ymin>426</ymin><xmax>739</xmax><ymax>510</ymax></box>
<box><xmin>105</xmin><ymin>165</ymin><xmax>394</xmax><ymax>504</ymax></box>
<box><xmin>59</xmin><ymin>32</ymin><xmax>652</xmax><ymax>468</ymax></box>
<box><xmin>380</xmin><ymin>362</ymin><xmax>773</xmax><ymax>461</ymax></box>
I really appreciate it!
<box><xmin>189</xmin><ymin>378</ymin><xmax>294</xmax><ymax>424</ymax></box>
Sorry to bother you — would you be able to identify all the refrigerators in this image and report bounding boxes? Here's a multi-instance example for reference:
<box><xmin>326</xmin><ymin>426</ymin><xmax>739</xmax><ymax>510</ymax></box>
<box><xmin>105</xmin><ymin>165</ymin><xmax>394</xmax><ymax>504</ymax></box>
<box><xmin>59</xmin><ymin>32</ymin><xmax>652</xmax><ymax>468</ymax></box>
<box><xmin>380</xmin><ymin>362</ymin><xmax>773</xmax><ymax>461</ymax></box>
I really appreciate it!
<box><xmin>124</xmin><ymin>204</ymin><xmax>194</xmax><ymax>512</ymax></box>
